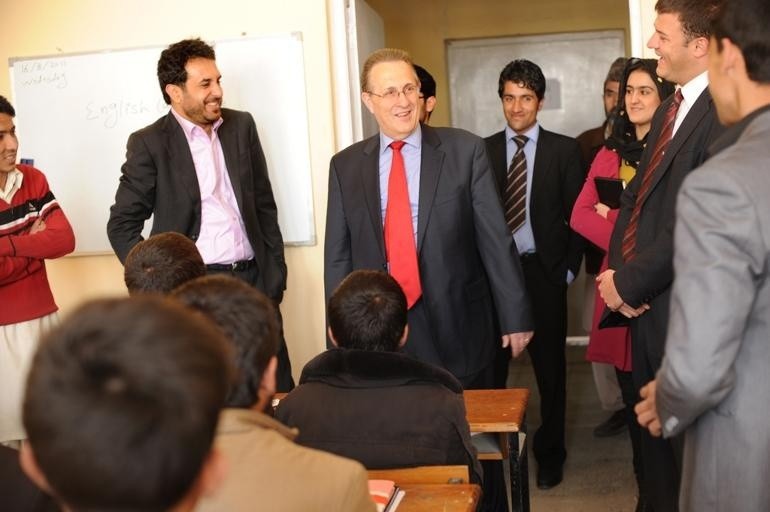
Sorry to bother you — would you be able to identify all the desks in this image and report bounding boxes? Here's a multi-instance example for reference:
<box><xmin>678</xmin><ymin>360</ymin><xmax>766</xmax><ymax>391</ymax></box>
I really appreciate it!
<box><xmin>269</xmin><ymin>384</ymin><xmax>533</xmax><ymax>512</ymax></box>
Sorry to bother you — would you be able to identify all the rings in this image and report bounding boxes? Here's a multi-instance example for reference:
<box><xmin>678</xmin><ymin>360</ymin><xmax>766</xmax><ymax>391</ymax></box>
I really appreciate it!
<box><xmin>522</xmin><ymin>337</ymin><xmax>530</xmax><ymax>344</ymax></box>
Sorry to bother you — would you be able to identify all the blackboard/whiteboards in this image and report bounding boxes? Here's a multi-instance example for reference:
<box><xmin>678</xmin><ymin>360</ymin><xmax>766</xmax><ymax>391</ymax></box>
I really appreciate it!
<box><xmin>8</xmin><ymin>30</ymin><xmax>318</xmax><ymax>258</ymax></box>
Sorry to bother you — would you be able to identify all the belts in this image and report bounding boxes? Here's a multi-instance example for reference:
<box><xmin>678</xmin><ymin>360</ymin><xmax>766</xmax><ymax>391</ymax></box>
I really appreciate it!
<box><xmin>205</xmin><ymin>257</ymin><xmax>255</xmax><ymax>271</ymax></box>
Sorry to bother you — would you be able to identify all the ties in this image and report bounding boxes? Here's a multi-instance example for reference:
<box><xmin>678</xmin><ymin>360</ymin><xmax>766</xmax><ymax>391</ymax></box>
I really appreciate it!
<box><xmin>384</xmin><ymin>140</ymin><xmax>423</xmax><ymax>310</ymax></box>
<box><xmin>621</xmin><ymin>88</ymin><xmax>684</xmax><ymax>266</ymax></box>
<box><xmin>503</xmin><ymin>135</ymin><xmax>530</xmax><ymax>234</ymax></box>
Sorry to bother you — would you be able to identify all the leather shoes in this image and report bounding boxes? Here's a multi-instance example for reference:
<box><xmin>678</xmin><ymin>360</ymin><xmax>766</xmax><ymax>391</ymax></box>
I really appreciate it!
<box><xmin>594</xmin><ymin>407</ymin><xmax>630</xmax><ymax>438</ymax></box>
<box><xmin>536</xmin><ymin>461</ymin><xmax>563</xmax><ymax>489</ymax></box>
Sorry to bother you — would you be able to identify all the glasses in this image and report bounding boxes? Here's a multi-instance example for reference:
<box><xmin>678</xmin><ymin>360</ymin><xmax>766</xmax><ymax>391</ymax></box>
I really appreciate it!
<box><xmin>367</xmin><ymin>83</ymin><xmax>421</xmax><ymax>100</ymax></box>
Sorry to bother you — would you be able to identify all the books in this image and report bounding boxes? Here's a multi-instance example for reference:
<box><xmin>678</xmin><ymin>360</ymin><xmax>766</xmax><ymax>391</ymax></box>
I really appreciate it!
<box><xmin>366</xmin><ymin>476</ymin><xmax>405</xmax><ymax>512</ymax></box>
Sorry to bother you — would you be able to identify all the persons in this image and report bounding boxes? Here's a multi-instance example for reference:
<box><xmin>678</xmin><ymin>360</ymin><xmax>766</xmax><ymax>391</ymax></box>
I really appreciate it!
<box><xmin>17</xmin><ymin>293</ymin><xmax>237</xmax><ymax>512</ymax></box>
<box><xmin>321</xmin><ymin>45</ymin><xmax>540</xmax><ymax>509</ymax></box>
<box><xmin>0</xmin><ymin>97</ymin><xmax>81</xmax><ymax>455</ymax></box>
<box><xmin>410</xmin><ymin>61</ymin><xmax>441</xmax><ymax>128</ymax></box>
<box><xmin>165</xmin><ymin>269</ymin><xmax>380</xmax><ymax>512</ymax></box>
<box><xmin>119</xmin><ymin>229</ymin><xmax>279</xmax><ymax>420</ymax></box>
<box><xmin>566</xmin><ymin>59</ymin><xmax>683</xmax><ymax>510</ymax></box>
<box><xmin>631</xmin><ymin>0</ymin><xmax>770</xmax><ymax>512</ymax></box>
<box><xmin>104</xmin><ymin>36</ymin><xmax>295</xmax><ymax>401</ymax></box>
<box><xmin>572</xmin><ymin>53</ymin><xmax>638</xmax><ymax>439</ymax></box>
<box><xmin>476</xmin><ymin>56</ymin><xmax>590</xmax><ymax>495</ymax></box>
<box><xmin>592</xmin><ymin>1</ymin><xmax>741</xmax><ymax>510</ymax></box>
<box><xmin>263</xmin><ymin>265</ymin><xmax>488</xmax><ymax>511</ymax></box>
